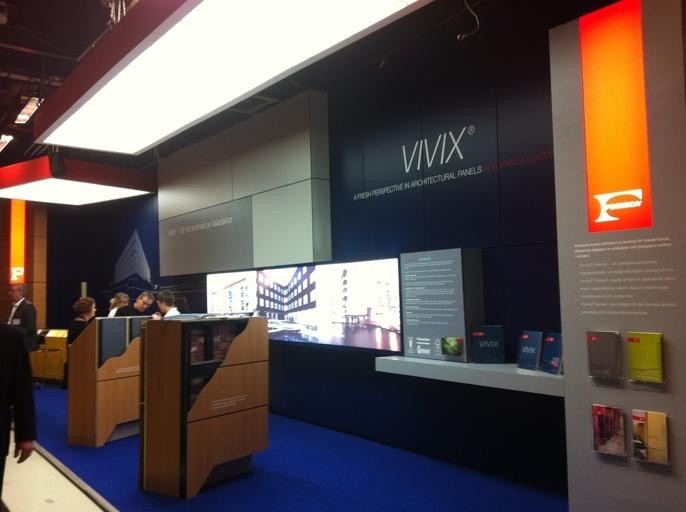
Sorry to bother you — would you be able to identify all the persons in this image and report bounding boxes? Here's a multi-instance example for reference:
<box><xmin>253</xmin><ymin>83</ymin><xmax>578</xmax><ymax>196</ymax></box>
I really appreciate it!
<box><xmin>61</xmin><ymin>296</ymin><xmax>97</xmax><ymax>389</ymax></box>
<box><xmin>0</xmin><ymin>321</ymin><xmax>38</xmax><ymax>512</ymax></box>
<box><xmin>151</xmin><ymin>290</ymin><xmax>180</xmax><ymax>320</ymax></box>
<box><xmin>6</xmin><ymin>284</ymin><xmax>38</xmax><ymax>351</ymax></box>
<box><xmin>114</xmin><ymin>290</ymin><xmax>155</xmax><ymax>316</ymax></box>
<box><xmin>107</xmin><ymin>291</ymin><xmax>130</xmax><ymax>317</ymax></box>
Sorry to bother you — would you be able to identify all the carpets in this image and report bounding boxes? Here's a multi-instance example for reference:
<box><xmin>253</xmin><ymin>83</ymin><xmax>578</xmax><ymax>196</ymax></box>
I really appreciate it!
<box><xmin>26</xmin><ymin>374</ymin><xmax>567</xmax><ymax>509</ymax></box>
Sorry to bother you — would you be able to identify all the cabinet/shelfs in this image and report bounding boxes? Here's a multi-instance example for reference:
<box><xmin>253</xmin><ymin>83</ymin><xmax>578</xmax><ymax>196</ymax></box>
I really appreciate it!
<box><xmin>66</xmin><ymin>315</ymin><xmax>142</xmax><ymax>450</ymax></box>
<box><xmin>138</xmin><ymin>310</ymin><xmax>269</xmax><ymax>502</ymax></box>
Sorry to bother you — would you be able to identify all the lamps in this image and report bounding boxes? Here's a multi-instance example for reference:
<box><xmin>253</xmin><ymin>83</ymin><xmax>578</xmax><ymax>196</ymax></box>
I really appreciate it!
<box><xmin>0</xmin><ymin>72</ymin><xmax>158</xmax><ymax>205</ymax></box>
<box><xmin>28</xmin><ymin>0</ymin><xmax>438</xmax><ymax>158</ymax></box>
